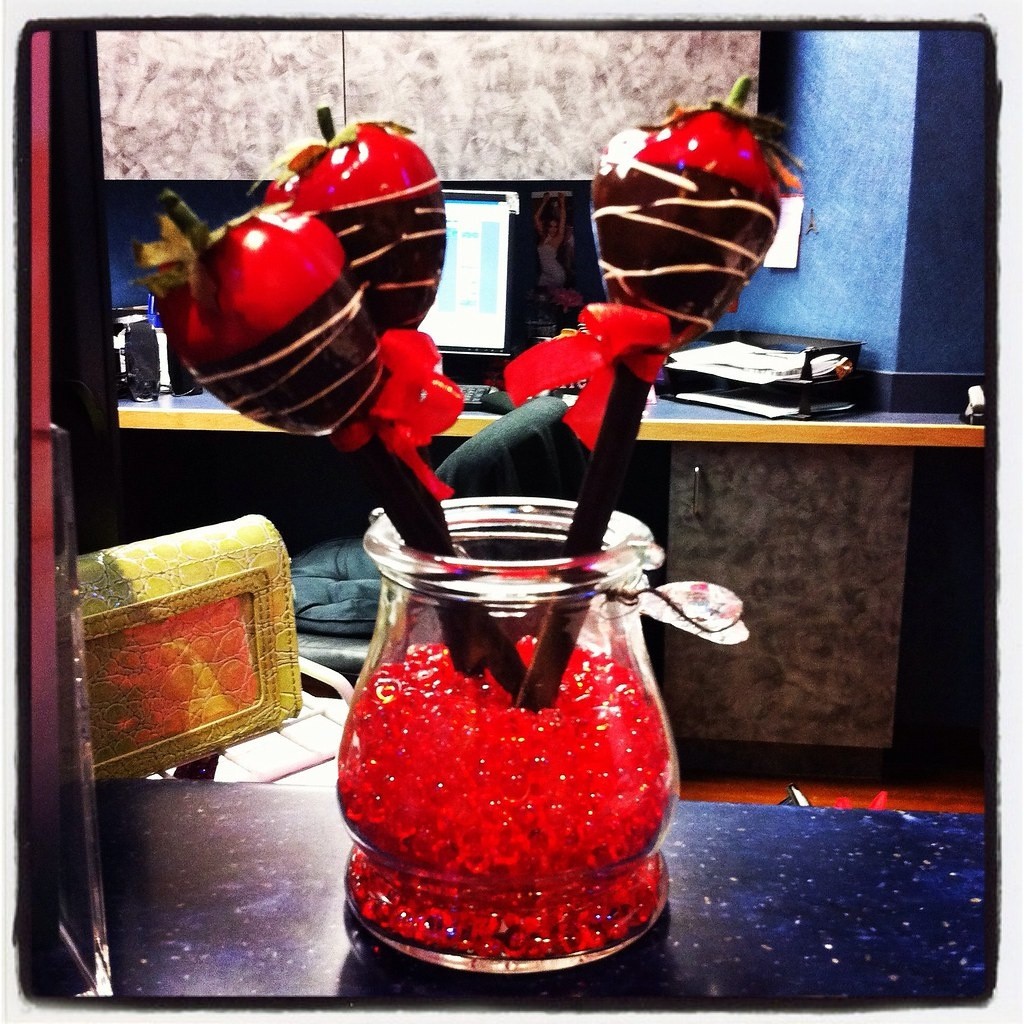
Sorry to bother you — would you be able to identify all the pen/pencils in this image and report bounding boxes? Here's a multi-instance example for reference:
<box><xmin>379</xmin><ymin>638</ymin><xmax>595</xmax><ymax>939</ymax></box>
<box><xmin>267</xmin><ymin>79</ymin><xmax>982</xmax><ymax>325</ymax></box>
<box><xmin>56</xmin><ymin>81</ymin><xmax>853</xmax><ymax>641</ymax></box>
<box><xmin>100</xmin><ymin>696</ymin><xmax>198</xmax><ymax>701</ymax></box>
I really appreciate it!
<box><xmin>144</xmin><ymin>291</ymin><xmax>158</xmax><ymax>328</ymax></box>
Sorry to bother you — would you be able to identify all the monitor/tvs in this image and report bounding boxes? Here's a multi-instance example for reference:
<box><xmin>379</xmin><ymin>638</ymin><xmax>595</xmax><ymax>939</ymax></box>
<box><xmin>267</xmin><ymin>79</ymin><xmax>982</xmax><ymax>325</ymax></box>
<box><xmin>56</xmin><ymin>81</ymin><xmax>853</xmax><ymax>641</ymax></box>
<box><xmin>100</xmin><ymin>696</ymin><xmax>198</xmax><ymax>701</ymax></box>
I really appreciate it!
<box><xmin>418</xmin><ymin>190</ymin><xmax>521</xmax><ymax>379</ymax></box>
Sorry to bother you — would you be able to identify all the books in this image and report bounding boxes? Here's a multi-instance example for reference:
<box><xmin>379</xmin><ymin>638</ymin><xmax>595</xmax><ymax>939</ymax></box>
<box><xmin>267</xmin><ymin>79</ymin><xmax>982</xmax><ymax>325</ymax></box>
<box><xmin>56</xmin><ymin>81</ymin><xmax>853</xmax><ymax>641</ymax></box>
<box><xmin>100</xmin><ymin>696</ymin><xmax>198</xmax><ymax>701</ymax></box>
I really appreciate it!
<box><xmin>666</xmin><ymin>341</ymin><xmax>850</xmax><ymax>418</ymax></box>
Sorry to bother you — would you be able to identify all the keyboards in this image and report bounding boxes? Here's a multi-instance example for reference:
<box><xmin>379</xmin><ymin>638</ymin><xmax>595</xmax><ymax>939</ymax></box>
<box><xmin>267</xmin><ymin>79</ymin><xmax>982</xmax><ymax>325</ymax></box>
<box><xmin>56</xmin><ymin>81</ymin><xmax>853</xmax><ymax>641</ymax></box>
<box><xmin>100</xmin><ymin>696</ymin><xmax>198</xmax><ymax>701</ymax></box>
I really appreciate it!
<box><xmin>455</xmin><ymin>385</ymin><xmax>499</xmax><ymax>409</ymax></box>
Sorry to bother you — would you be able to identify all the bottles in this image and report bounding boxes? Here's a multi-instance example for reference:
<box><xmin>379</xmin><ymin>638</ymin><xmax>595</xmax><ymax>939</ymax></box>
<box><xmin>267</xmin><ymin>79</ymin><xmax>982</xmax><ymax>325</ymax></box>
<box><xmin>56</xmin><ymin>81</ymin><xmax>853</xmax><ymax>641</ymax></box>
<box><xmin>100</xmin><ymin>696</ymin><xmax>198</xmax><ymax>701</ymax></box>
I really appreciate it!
<box><xmin>336</xmin><ymin>496</ymin><xmax>682</xmax><ymax>974</ymax></box>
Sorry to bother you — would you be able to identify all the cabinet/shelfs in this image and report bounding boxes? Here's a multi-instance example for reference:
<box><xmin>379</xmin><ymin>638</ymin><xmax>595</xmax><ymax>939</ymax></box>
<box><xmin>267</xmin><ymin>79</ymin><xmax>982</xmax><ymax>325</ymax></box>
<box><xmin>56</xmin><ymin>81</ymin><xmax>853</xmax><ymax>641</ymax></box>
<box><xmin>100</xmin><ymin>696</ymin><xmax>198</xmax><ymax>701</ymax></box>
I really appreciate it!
<box><xmin>91</xmin><ymin>30</ymin><xmax>763</xmax><ymax>190</ymax></box>
<box><xmin>119</xmin><ymin>370</ymin><xmax>986</xmax><ymax>784</ymax></box>
